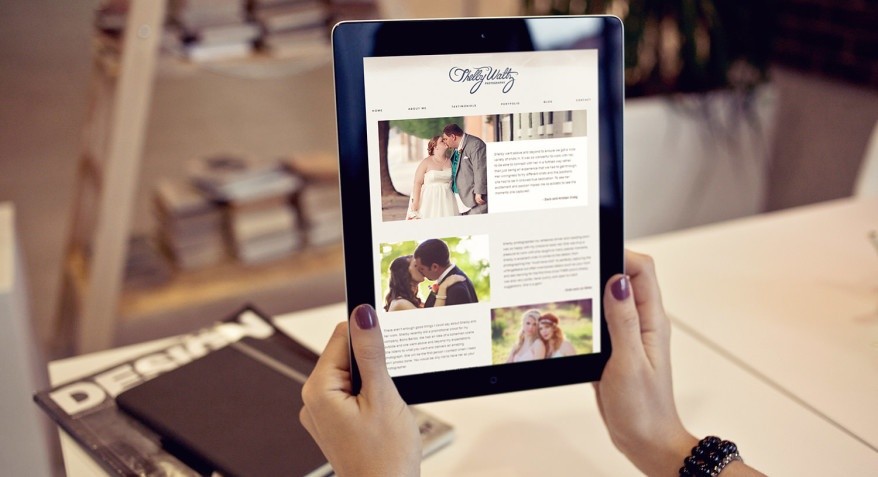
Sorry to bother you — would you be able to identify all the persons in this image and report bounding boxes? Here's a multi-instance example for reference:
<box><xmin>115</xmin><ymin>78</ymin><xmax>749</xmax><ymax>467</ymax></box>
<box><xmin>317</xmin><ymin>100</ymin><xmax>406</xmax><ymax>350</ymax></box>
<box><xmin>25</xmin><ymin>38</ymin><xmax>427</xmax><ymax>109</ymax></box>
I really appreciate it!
<box><xmin>507</xmin><ymin>310</ymin><xmax>546</xmax><ymax>365</ymax></box>
<box><xmin>538</xmin><ymin>313</ymin><xmax>575</xmax><ymax>361</ymax></box>
<box><xmin>409</xmin><ymin>136</ymin><xmax>460</xmax><ymax>220</ymax></box>
<box><xmin>444</xmin><ymin>126</ymin><xmax>487</xmax><ymax>214</ymax></box>
<box><xmin>415</xmin><ymin>239</ymin><xmax>478</xmax><ymax>309</ymax></box>
<box><xmin>302</xmin><ymin>248</ymin><xmax>769</xmax><ymax>477</ymax></box>
<box><xmin>385</xmin><ymin>255</ymin><xmax>466</xmax><ymax>312</ymax></box>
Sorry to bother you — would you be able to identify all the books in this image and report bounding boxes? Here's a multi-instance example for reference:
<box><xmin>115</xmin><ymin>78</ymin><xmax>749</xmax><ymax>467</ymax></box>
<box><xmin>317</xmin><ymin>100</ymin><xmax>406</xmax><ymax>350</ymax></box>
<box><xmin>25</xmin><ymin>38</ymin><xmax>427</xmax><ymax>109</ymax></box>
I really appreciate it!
<box><xmin>36</xmin><ymin>303</ymin><xmax>456</xmax><ymax>477</ymax></box>
<box><xmin>77</xmin><ymin>0</ymin><xmax>343</xmax><ymax>285</ymax></box>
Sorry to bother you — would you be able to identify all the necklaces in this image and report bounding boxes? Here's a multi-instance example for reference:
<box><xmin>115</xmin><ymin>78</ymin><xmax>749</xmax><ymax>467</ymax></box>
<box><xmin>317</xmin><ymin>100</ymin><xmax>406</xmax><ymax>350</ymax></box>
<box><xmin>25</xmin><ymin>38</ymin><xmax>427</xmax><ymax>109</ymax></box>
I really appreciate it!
<box><xmin>432</xmin><ymin>155</ymin><xmax>447</xmax><ymax>165</ymax></box>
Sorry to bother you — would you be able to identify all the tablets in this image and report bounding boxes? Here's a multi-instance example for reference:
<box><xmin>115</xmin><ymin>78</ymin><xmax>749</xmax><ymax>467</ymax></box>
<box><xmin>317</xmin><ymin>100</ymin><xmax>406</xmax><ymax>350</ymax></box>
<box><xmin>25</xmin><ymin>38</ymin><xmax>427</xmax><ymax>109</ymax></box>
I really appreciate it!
<box><xmin>332</xmin><ymin>14</ymin><xmax>627</xmax><ymax>407</ymax></box>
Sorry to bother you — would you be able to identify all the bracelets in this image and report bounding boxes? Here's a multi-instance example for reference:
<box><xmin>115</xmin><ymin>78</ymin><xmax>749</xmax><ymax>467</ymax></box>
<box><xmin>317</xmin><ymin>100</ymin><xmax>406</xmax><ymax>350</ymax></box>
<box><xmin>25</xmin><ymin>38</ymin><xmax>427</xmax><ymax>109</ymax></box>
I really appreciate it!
<box><xmin>677</xmin><ymin>436</ymin><xmax>745</xmax><ymax>477</ymax></box>
<box><xmin>435</xmin><ymin>295</ymin><xmax>447</xmax><ymax>299</ymax></box>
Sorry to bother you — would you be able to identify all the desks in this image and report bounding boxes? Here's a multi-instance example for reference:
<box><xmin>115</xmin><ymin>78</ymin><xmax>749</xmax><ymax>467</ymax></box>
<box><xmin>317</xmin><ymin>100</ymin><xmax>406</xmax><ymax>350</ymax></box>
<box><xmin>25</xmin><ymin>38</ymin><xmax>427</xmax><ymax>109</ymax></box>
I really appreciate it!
<box><xmin>50</xmin><ymin>194</ymin><xmax>878</xmax><ymax>477</ymax></box>
